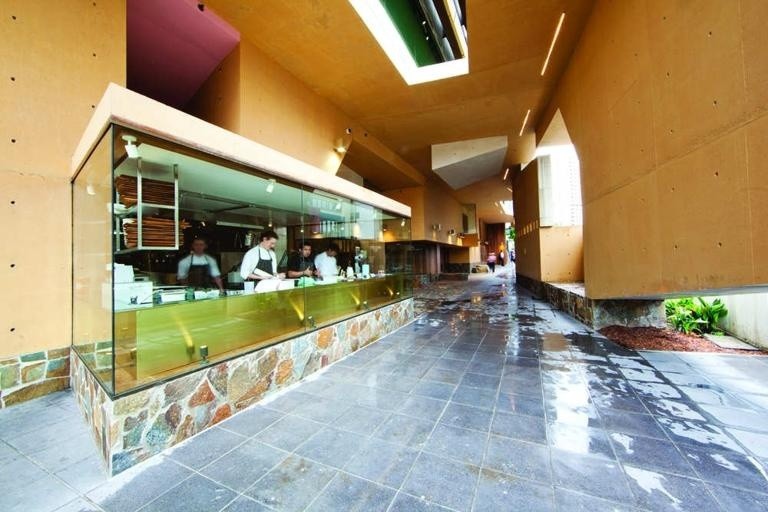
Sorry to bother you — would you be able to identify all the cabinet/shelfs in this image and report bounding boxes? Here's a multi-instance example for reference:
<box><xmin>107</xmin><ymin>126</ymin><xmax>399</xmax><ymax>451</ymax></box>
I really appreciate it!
<box><xmin>112</xmin><ymin>164</ymin><xmax>181</xmax><ymax>252</ymax></box>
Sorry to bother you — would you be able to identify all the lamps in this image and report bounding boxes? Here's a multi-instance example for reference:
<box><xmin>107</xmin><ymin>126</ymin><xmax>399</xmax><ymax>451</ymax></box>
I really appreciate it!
<box><xmin>308</xmin><ymin>316</ymin><xmax>317</xmax><ymax>328</ymax></box>
<box><xmin>200</xmin><ymin>346</ymin><xmax>210</xmax><ymax>365</ymax></box>
<box><xmin>363</xmin><ymin>301</ymin><xmax>370</xmax><ymax>311</ymax></box>
<box><xmin>333</xmin><ymin>138</ymin><xmax>346</xmax><ymax>154</ymax></box>
<box><xmin>396</xmin><ymin>292</ymin><xmax>401</xmax><ymax>299</ymax></box>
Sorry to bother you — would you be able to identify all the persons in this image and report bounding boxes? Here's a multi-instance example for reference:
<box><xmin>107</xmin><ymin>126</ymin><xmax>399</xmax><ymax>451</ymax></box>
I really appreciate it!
<box><xmin>491</xmin><ymin>252</ymin><xmax>496</xmax><ymax>272</ymax></box>
<box><xmin>500</xmin><ymin>250</ymin><xmax>505</xmax><ymax>266</ymax></box>
<box><xmin>511</xmin><ymin>250</ymin><xmax>516</xmax><ymax>262</ymax></box>
<box><xmin>286</xmin><ymin>243</ymin><xmax>321</xmax><ymax>278</ymax></box>
<box><xmin>240</xmin><ymin>230</ymin><xmax>286</xmax><ymax>280</ymax></box>
<box><xmin>178</xmin><ymin>238</ymin><xmax>224</xmax><ymax>293</ymax></box>
<box><xmin>314</xmin><ymin>244</ymin><xmax>340</xmax><ymax>279</ymax></box>
<box><xmin>487</xmin><ymin>252</ymin><xmax>491</xmax><ymax>273</ymax></box>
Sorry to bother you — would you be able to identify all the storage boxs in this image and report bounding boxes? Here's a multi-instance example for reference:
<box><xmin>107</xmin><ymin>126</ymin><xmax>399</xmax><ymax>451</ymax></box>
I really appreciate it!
<box><xmin>101</xmin><ymin>281</ymin><xmax>152</xmax><ymax>311</ymax></box>
